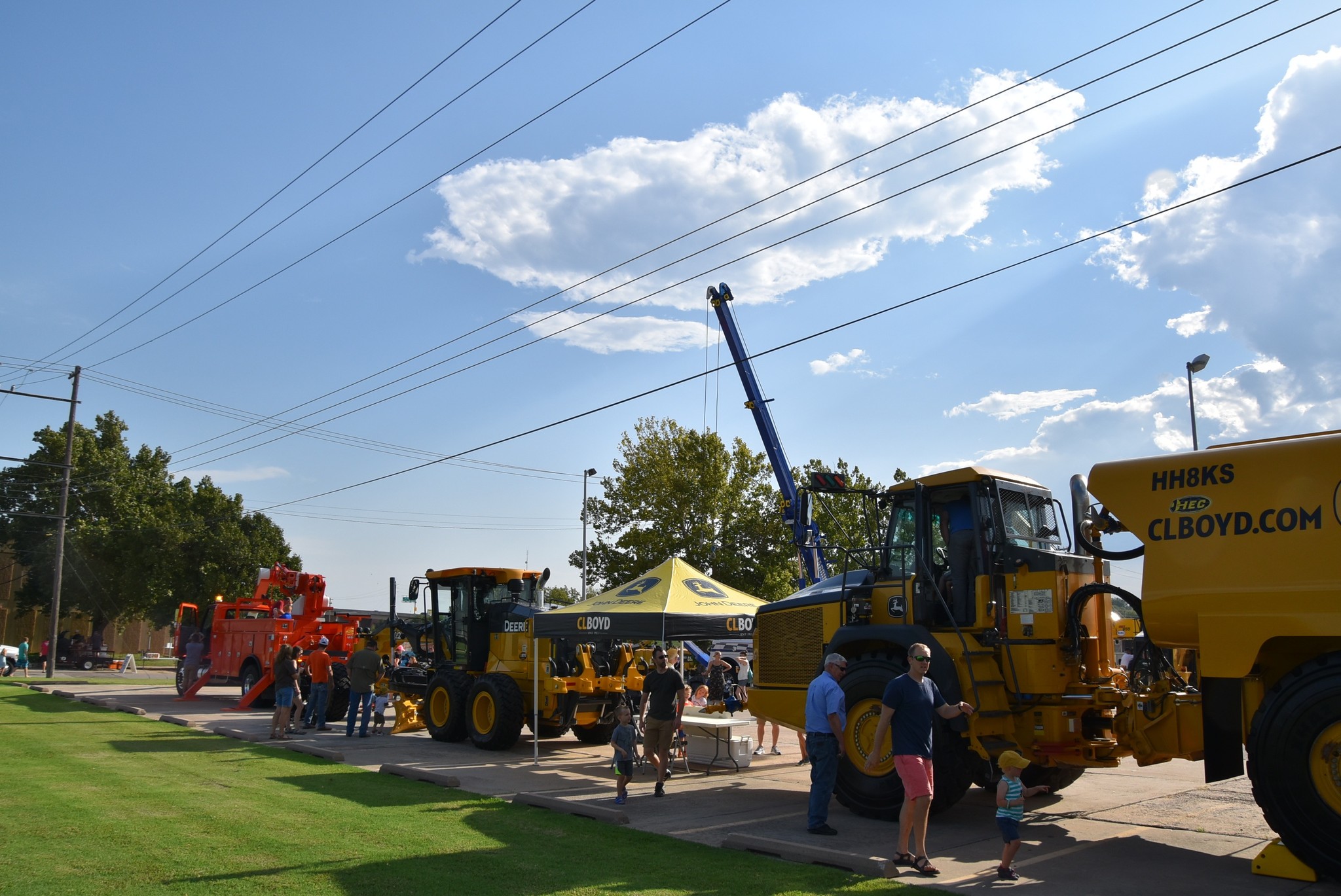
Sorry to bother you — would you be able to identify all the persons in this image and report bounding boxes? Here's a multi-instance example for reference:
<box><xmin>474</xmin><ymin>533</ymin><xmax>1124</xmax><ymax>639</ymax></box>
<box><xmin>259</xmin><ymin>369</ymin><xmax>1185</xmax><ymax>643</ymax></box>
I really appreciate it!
<box><xmin>795</xmin><ymin>731</ymin><xmax>811</xmax><ymax>766</ymax></box>
<box><xmin>753</xmin><ymin>717</ymin><xmax>781</xmax><ymax>755</ymax></box>
<box><xmin>995</xmin><ymin>750</ymin><xmax>1050</xmax><ymax>881</ymax></box>
<box><xmin>701</xmin><ymin>651</ymin><xmax>732</xmax><ymax>705</ymax></box>
<box><xmin>936</xmin><ymin>499</ymin><xmax>979</xmax><ymax>627</ymax></box>
<box><xmin>805</xmin><ymin>653</ymin><xmax>848</xmax><ymax>836</ymax></box>
<box><xmin>268</xmin><ymin>597</ymin><xmax>292</xmax><ymax>619</ymax></box>
<box><xmin>639</xmin><ymin>646</ymin><xmax>685</xmax><ymax>798</ymax></box>
<box><xmin>1120</xmin><ymin>647</ymin><xmax>1134</xmax><ymax>669</ymax></box>
<box><xmin>735</xmin><ymin>650</ymin><xmax>749</xmax><ymax>703</ymax></box>
<box><xmin>611</xmin><ymin>705</ymin><xmax>640</xmax><ymax>805</ymax></box>
<box><xmin>269</xmin><ymin>637</ymin><xmax>434</xmax><ymax>740</ymax></box>
<box><xmin>864</xmin><ymin>643</ymin><xmax>975</xmax><ymax>875</ymax></box>
<box><xmin>651</xmin><ymin>642</ymin><xmax>681</xmax><ymax>669</ymax></box>
<box><xmin>181</xmin><ymin>627</ymin><xmax>205</xmax><ymax>698</ymax></box>
<box><xmin>0</xmin><ymin>629</ymin><xmax>101</xmax><ymax>677</ymax></box>
<box><xmin>676</xmin><ymin>685</ymin><xmax>709</xmax><ymax>757</ymax></box>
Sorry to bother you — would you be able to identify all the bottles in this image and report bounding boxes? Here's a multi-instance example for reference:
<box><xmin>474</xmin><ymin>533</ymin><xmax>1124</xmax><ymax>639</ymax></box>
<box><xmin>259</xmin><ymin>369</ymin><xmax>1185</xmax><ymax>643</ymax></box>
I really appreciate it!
<box><xmin>71</xmin><ymin>638</ymin><xmax>76</xmax><ymax>645</ymax></box>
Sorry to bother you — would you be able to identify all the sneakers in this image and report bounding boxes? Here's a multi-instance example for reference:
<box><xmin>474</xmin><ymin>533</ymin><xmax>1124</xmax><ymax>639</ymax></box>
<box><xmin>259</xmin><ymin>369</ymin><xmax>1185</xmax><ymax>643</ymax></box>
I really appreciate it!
<box><xmin>997</xmin><ymin>866</ymin><xmax>1019</xmax><ymax>878</ymax></box>
<box><xmin>771</xmin><ymin>746</ymin><xmax>782</xmax><ymax>755</ymax></box>
<box><xmin>657</xmin><ymin>769</ymin><xmax>671</xmax><ymax>782</ymax></box>
<box><xmin>754</xmin><ymin>746</ymin><xmax>765</xmax><ymax>755</ymax></box>
<box><xmin>654</xmin><ymin>782</ymin><xmax>665</xmax><ymax>797</ymax></box>
<box><xmin>998</xmin><ymin>868</ymin><xmax>1019</xmax><ymax>880</ymax></box>
<box><xmin>796</xmin><ymin>760</ymin><xmax>807</xmax><ymax>766</ymax></box>
<box><xmin>804</xmin><ymin>755</ymin><xmax>810</xmax><ymax>764</ymax></box>
<box><xmin>807</xmin><ymin>823</ymin><xmax>838</xmax><ymax>835</ymax></box>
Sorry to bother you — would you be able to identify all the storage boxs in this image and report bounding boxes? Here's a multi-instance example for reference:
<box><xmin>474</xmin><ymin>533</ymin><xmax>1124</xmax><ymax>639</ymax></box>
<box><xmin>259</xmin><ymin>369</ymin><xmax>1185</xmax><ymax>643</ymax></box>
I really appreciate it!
<box><xmin>108</xmin><ymin>660</ymin><xmax>125</xmax><ymax>669</ymax></box>
<box><xmin>682</xmin><ymin>706</ymin><xmax>753</xmax><ymax>768</ymax></box>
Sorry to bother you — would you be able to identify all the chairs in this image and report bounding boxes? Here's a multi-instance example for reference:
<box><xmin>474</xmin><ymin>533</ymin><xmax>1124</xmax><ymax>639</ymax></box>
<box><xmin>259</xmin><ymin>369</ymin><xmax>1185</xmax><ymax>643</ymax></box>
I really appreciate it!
<box><xmin>610</xmin><ymin>718</ymin><xmax>691</xmax><ymax>774</ymax></box>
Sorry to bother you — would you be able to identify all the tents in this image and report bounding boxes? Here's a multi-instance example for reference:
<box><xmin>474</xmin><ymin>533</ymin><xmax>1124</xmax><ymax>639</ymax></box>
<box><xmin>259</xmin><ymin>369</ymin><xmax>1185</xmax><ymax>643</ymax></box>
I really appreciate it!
<box><xmin>532</xmin><ymin>557</ymin><xmax>772</xmax><ymax>766</ymax></box>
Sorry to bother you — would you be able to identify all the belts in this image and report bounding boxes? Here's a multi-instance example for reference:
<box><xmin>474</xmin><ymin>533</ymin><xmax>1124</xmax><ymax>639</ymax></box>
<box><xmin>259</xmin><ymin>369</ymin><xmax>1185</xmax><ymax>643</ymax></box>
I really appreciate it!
<box><xmin>806</xmin><ymin>732</ymin><xmax>836</xmax><ymax>737</ymax></box>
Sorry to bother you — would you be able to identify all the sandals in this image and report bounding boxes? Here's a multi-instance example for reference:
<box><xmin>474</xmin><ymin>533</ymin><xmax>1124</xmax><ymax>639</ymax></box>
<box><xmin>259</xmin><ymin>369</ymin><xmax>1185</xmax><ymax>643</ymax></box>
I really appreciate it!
<box><xmin>912</xmin><ymin>856</ymin><xmax>941</xmax><ymax>874</ymax></box>
<box><xmin>622</xmin><ymin>790</ymin><xmax>628</xmax><ymax>798</ymax></box>
<box><xmin>285</xmin><ymin>727</ymin><xmax>294</xmax><ymax>733</ymax></box>
<box><xmin>270</xmin><ymin>733</ymin><xmax>278</xmax><ymax>739</ymax></box>
<box><xmin>616</xmin><ymin>796</ymin><xmax>625</xmax><ymax>805</ymax></box>
<box><xmin>892</xmin><ymin>852</ymin><xmax>914</xmax><ymax>865</ymax></box>
<box><xmin>294</xmin><ymin>728</ymin><xmax>307</xmax><ymax>734</ymax></box>
<box><xmin>277</xmin><ymin>734</ymin><xmax>293</xmax><ymax>739</ymax></box>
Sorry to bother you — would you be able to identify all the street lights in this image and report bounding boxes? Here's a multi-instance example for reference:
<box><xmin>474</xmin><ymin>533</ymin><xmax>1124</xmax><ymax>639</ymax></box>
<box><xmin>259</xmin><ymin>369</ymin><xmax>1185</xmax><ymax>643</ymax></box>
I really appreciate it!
<box><xmin>582</xmin><ymin>468</ymin><xmax>598</xmax><ymax>601</ymax></box>
<box><xmin>1182</xmin><ymin>353</ymin><xmax>1210</xmax><ymax>452</ymax></box>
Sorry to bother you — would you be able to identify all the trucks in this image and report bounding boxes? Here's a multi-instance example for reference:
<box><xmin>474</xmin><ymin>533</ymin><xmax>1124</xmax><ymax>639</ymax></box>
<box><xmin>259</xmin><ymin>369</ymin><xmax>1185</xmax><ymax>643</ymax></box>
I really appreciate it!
<box><xmin>55</xmin><ymin>630</ymin><xmax>115</xmax><ymax>671</ymax></box>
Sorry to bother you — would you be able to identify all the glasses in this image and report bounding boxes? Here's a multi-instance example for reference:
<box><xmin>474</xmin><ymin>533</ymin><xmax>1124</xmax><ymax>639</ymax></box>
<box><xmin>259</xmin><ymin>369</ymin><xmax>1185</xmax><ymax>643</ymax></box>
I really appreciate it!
<box><xmin>910</xmin><ymin>656</ymin><xmax>931</xmax><ymax>662</ymax></box>
<box><xmin>833</xmin><ymin>663</ymin><xmax>846</xmax><ymax>671</ymax></box>
<box><xmin>656</xmin><ymin>655</ymin><xmax>669</xmax><ymax>659</ymax></box>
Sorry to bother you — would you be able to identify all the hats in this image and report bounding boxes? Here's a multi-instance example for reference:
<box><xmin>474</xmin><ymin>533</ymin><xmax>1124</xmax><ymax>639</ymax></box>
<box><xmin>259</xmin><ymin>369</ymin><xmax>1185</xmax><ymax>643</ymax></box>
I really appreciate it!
<box><xmin>380</xmin><ymin>687</ymin><xmax>390</xmax><ymax>694</ymax></box>
<box><xmin>367</xmin><ymin>640</ymin><xmax>379</xmax><ymax>651</ymax></box>
<box><xmin>997</xmin><ymin>750</ymin><xmax>1031</xmax><ymax>768</ymax></box>
<box><xmin>319</xmin><ymin>637</ymin><xmax>329</xmax><ymax>645</ymax></box>
<box><xmin>395</xmin><ymin>645</ymin><xmax>406</xmax><ymax>652</ymax></box>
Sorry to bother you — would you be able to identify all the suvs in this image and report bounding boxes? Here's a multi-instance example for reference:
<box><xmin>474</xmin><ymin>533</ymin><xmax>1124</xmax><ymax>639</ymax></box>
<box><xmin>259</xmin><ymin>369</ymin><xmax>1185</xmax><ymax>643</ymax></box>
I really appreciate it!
<box><xmin>0</xmin><ymin>644</ymin><xmax>19</xmax><ymax>677</ymax></box>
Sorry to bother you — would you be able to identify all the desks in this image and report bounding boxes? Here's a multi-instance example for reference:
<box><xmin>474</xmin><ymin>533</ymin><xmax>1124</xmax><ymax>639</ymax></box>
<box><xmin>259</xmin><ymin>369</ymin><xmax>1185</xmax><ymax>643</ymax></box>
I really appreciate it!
<box><xmin>632</xmin><ymin>714</ymin><xmax>750</xmax><ymax>776</ymax></box>
<box><xmin>141</xmin><ymin>657</ymin><xmax>179</xmax><ymax>670</ymax></box>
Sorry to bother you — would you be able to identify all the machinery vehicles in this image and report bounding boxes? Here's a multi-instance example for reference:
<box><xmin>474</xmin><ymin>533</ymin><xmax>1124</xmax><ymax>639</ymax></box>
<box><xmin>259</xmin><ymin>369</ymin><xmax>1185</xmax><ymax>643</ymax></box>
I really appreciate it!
<box><xmin>353</xmin><ymin>564</ymin><xmax>755</xmax><ymax>750</ymax></box>
<box><xmin>170</xmin><ymin>562</ymin><xmax>375</xmax><ymax>721</ymax></box>
<box><xmin>704</xmin><ymin>281</ymin><xmax>829</xmax><ymax>591</ymax></box>
<box><xmin>748</xmin><ymin>429</ymin><xmax>1341</xmax><ymax>883</ymax></box>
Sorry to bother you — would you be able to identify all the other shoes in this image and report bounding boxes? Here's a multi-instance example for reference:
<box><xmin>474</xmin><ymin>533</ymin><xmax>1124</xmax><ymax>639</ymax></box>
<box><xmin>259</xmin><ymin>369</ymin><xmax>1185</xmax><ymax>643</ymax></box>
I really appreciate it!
<box><xmin>302</xmin><ymin>723</ymin><xmax>316</xmax><ymax>728</ymax></box>
<box><xmin>372</xmin><ymin>730</ymin><xmax>378</xmax><ymax>734</ymax></box>
<box><xmin>378</xmin><ymin>732</ymin><xmax>385</xmax><ymax>736</ymax></box>
<box><xmin>323</xmin><ymin>726</ymin><xmax>332</xmax><ymax>730</ymax></box>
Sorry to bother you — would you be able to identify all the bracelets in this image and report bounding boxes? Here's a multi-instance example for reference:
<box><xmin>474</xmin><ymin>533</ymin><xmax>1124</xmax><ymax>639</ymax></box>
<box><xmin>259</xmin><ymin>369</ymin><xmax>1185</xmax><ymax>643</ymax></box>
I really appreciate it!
<box><xmin>958</xmin><ymin>704</ymin><xmax>962</xmax><ymax>711</ymax></box>
<box><xmin>1007</xmin><ymin>800</ymin><xmax>1010</xmax><ymax>810</ymax></box>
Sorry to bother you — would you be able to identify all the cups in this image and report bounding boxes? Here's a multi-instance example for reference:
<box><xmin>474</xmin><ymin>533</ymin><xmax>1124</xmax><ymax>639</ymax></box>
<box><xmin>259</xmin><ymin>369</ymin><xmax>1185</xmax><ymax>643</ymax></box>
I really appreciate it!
<box><xmin>144</xmin><ymin>657</ymin><xmax>146</xmax><ymax>658</ymax></box>
